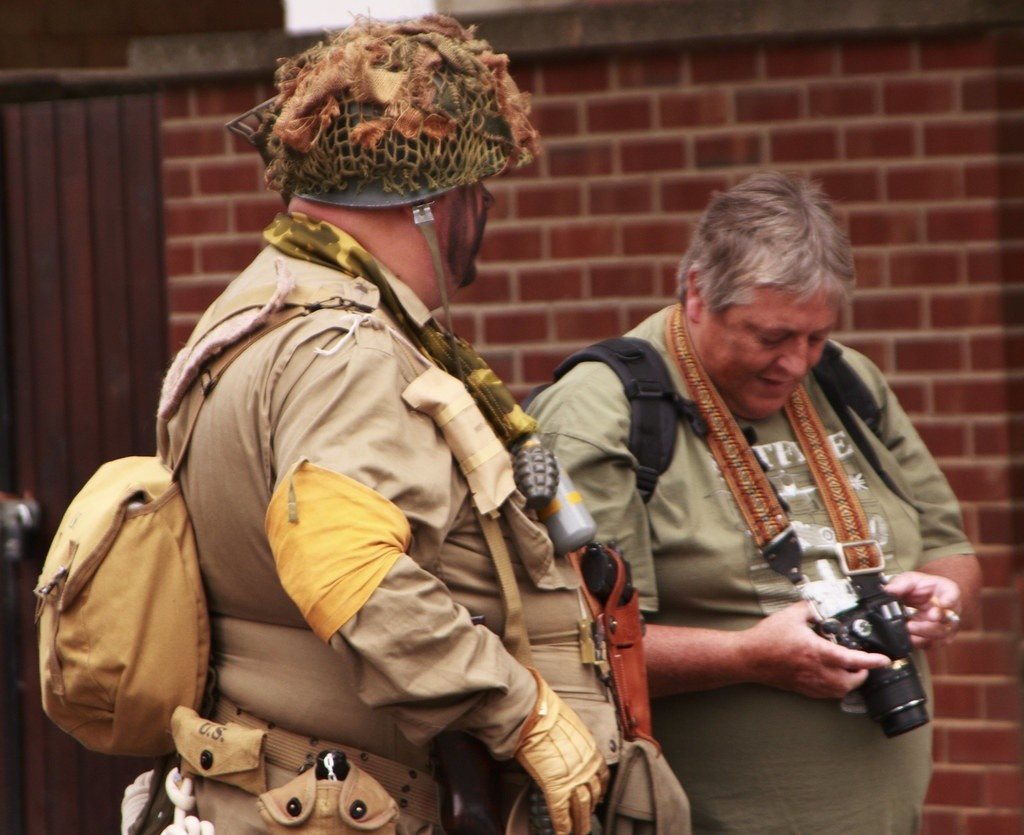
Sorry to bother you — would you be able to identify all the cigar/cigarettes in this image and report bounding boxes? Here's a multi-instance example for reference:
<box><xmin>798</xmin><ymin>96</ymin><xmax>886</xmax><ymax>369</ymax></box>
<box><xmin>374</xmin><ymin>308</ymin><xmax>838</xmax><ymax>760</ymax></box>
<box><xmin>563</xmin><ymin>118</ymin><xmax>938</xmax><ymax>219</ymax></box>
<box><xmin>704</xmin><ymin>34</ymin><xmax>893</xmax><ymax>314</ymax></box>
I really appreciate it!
<box><xmin>929</xmin><ymin>596</ymin><xmax>961</xmax><ymax>623</ymax></box>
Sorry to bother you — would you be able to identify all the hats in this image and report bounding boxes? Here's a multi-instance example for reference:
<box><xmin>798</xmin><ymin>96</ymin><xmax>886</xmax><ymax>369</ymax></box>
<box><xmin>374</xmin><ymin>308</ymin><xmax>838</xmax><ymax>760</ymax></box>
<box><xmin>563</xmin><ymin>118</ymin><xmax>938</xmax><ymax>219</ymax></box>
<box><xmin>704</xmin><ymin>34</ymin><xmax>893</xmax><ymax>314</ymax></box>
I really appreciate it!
<box><xmin>223</xmin><ymin>10</ymin><xmax>545</xmax><ymax>209</ymax></box>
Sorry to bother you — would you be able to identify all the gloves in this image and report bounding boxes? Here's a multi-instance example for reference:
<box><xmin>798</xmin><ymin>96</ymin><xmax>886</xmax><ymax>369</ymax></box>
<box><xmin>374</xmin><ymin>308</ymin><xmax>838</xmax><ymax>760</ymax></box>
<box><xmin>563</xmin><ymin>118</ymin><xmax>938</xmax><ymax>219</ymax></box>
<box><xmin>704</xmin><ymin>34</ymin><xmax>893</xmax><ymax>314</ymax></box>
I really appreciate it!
<box><xmin>516</xmin><ymin>666</ymin><xmax>611</xmax><ymax>835</ymax></box>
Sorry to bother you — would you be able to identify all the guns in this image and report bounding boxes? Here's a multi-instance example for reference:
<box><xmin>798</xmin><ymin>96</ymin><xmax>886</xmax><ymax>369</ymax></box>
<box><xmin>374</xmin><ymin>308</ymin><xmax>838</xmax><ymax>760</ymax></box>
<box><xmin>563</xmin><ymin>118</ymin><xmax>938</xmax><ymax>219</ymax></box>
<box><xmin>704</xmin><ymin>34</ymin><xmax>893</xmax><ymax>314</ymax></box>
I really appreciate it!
<box><xmin>579</xmin><ymin>536</ymin><xmax>637</xmax><ymax>610</ymax></box>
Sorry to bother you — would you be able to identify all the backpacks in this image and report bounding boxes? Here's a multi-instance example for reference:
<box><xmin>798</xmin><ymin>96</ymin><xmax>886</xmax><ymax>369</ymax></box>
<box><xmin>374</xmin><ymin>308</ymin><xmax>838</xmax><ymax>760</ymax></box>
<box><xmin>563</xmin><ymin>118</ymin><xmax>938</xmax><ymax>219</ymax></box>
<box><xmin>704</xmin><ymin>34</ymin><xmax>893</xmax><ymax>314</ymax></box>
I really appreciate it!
<box><xmin>32</xmin><ymin>300</ymin><xmax>441</xmax><ymax>757</ymax></box>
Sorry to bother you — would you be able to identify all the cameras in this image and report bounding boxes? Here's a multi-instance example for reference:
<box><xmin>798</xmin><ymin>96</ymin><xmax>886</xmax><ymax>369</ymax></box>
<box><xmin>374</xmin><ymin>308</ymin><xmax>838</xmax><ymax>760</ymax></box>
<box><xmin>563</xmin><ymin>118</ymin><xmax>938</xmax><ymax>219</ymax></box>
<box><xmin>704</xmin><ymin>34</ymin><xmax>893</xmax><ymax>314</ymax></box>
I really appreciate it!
<box><xmin>810</xmin><ymin>590</ymin><xmax>930</xmax><ymax>738</ymax></box>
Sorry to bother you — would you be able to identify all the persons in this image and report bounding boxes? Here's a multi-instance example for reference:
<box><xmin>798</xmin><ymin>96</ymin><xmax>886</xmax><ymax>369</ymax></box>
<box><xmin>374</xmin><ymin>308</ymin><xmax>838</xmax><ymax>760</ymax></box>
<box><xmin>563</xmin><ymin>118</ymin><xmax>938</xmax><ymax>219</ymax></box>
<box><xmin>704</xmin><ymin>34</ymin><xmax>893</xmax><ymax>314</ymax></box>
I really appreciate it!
<box><xmin>32</xmin><ymin>4</ymin><xmax>690</xmax><ymax>835</ymax></box>
<box><xmin>526</xmin><ymin>169</ymin><xmax>981</xmax><ymax>835</ymax></box>
